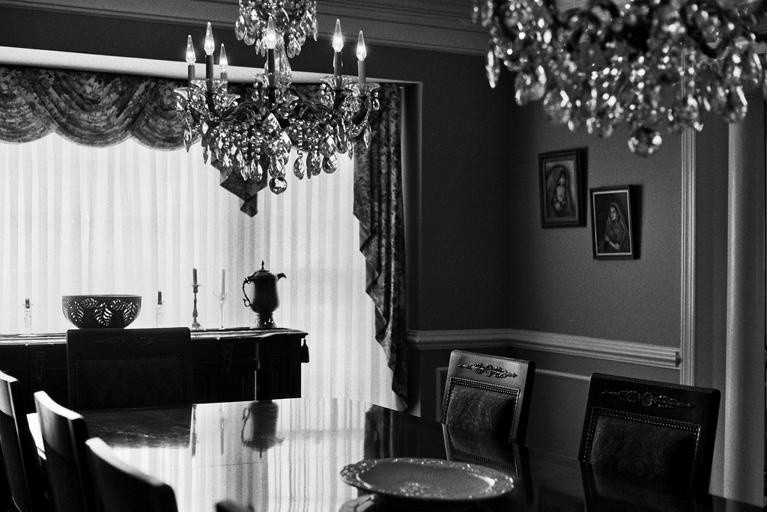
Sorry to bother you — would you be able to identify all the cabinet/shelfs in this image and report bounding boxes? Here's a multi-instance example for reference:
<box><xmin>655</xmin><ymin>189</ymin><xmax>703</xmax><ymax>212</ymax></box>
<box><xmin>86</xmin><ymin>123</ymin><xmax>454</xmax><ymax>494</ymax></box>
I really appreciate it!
<box><xmin>0</xmin><ymin>330</ymin><xmax>306</xmax><ymax>404</ymax></box>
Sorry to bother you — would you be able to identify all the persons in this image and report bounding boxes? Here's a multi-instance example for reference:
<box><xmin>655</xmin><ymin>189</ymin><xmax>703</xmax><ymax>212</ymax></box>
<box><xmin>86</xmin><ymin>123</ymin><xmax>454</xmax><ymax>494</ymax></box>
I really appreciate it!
<box><xmin>599</xmin><ymin>203</ymin><xmax>629</xmax><ymax>252</ymax></box>
<box><xmin>546</xmin><ymin>166</ymin><xmax>574</xmax><ymax>219</ymax></box>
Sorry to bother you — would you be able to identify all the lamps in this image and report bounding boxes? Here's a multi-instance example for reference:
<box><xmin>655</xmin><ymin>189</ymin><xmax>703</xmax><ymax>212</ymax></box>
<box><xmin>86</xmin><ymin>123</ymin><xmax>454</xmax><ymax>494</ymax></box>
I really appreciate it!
<box><xmin>169</xmin><ymin>0</ymin><xmax>377</xmax><ymax>196</ymax></box>
<box><xmin>470</xmin><ymin>0</ymin><xmax>765</xmax><ymax>157</ymax></box>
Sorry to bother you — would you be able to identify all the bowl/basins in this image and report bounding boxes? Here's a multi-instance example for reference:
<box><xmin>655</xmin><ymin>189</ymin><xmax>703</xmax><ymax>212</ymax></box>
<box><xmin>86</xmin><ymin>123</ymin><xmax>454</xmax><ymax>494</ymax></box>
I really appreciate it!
<box><xmin>62</xmin><ymin>295</ymin><xmax>141</xmax><ymax>330</ymax></box>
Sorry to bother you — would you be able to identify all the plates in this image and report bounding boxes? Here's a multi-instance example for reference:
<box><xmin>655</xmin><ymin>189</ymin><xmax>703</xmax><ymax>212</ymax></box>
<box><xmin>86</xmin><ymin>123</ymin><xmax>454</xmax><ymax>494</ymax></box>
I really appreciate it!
<box><xmin>338</xmin><ymin>454</ymin><xmax>515</xmax><ymax>501</ymax></box>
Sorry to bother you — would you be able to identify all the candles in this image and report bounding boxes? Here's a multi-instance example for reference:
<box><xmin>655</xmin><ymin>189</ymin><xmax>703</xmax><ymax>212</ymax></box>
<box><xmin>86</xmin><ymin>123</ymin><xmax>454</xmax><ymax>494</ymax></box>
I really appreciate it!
<box><xmin>192</xmin><ymin>268</ymin><xmax>198</xmax><ymax>284</ymax></box>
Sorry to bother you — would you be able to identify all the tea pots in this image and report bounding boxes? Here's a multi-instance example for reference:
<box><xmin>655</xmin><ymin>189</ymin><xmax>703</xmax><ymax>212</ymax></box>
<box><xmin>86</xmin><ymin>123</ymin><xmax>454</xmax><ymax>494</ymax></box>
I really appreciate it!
<box><xmin>240</xmin><ymin>259</ymin><xmax>286</xmax><ymax>327</ymax></box>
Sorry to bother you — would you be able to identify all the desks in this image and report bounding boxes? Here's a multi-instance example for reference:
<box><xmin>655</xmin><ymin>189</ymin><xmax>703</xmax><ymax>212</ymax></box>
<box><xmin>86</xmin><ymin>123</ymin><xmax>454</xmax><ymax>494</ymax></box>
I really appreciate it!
<box><xmin>83</xmin><ymin>401</ymin><xmax>762</xmax><ymax>510</ymax></box>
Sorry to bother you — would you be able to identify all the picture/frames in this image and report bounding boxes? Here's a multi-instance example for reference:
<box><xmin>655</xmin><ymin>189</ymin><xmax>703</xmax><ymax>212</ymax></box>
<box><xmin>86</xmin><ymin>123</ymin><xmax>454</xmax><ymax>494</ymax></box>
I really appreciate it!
<box><xmin>535</xmin><ymin>146</ymin><xmax>585</xmax><ymax>226</ymax></box>
<box><xmin>590</xmin><ymin>185</ymin><xmax>639</xmax><ymax>260</ymax></box>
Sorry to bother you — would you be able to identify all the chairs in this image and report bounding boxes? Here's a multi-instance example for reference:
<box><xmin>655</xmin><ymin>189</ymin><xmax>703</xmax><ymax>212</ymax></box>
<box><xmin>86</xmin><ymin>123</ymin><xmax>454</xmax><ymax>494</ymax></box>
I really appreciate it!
<box><xmin>87</xmin><ymin>436</ymin><xmax>175</xmax><ymax>512</ymax></box>
<box><xmin>66</xmin><ymin>328</ymin><xmax>190</xmax><ymax>405</ymax></box>
<box><xmin>33</xmin><ymin>389</ymin><xmax>88</xmax><ymax>511</ymax></box>
<box><xmin>575</xmin><ymin>372</ymin><xmax>721</xmax><ymax>507</ymax></box>
<box><xmin>1</xmin><ymin>374</ymin><xmax>43</xmax><ymax>511</ymax></box>
<box><xmin>441</xmin><ymin>349</ymin><xmax>531</xmax><ymax>494</ymax></box>
